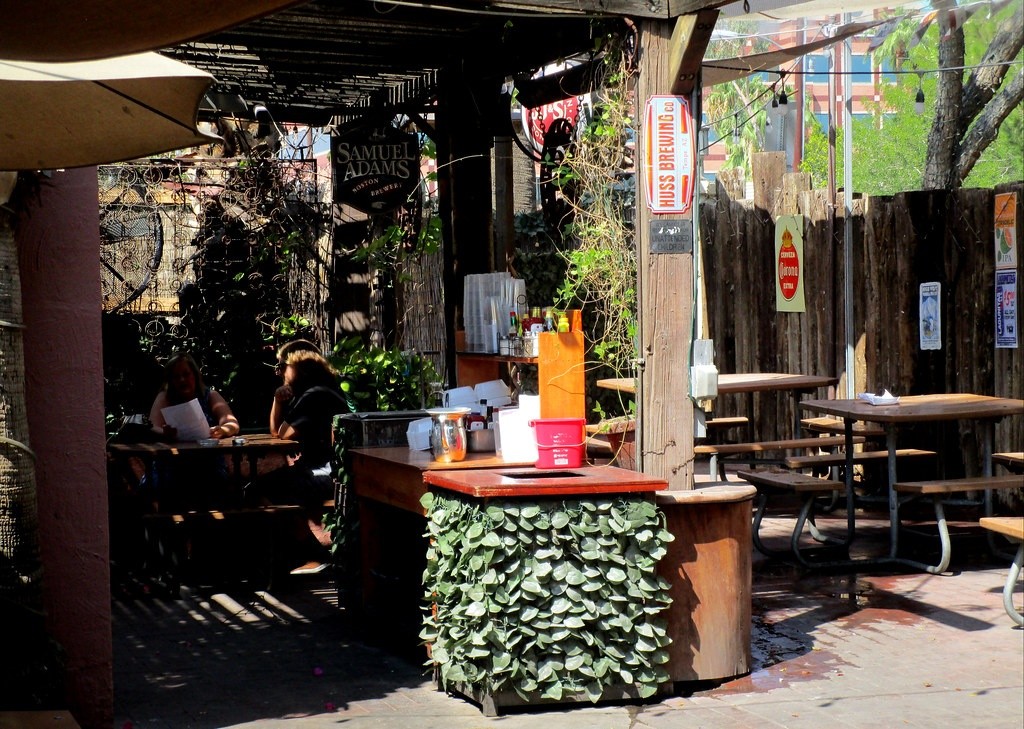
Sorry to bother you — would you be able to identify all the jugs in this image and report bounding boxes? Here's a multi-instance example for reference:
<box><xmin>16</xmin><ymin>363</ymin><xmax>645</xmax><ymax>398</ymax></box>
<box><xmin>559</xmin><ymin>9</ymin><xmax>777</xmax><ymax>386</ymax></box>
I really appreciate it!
<box><xmin>432</xmin><ymin>415</ymin><xmax>467</xmax><ymax>464</ymax></box>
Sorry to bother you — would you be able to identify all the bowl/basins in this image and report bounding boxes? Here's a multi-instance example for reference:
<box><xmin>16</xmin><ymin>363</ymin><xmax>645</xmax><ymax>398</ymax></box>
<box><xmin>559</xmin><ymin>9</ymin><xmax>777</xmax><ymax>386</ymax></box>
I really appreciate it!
<box><xmin>464</xmin><ymin>429</ymin><xmax>496</xmax><ymax>451</ymax></box>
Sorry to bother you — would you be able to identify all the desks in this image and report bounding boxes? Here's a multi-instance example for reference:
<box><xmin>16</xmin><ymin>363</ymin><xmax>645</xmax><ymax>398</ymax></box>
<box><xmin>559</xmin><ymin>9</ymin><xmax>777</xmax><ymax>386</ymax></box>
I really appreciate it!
<box><xmin>107</xmin><ymin>433</ymin><xmax>300</xmax><ymax>512</ymax></box>
<box><xmin>798</xmin><ymin>393</ymin><xmax>1024</xmax><ymax>565</ymax></box>
<box><xmin>596</xmin><ymin>372</ymin><xmax>839</xmax><ymax>501</ymax></box>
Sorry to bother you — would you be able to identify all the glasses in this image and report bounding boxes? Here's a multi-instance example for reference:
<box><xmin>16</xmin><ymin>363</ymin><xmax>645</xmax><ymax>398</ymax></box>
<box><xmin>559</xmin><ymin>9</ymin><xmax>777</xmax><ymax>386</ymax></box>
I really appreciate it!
<box><xmin>279</xmin><ymin>362</ymin><xmax>290</xmax><ymax>372</ymax></box>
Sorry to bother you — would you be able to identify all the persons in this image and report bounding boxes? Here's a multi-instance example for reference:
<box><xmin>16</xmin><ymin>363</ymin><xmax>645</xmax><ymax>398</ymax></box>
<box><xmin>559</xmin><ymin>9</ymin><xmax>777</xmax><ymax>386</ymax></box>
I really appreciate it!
<box><xmin>241</xmin><ymin>340</ymin><xmax>352</xmax><ymax>579</ymax></box>
<box><xmin>148</xmin><ymin>353</ymin><xmax>239</xmax><ymax>572</ymax></box>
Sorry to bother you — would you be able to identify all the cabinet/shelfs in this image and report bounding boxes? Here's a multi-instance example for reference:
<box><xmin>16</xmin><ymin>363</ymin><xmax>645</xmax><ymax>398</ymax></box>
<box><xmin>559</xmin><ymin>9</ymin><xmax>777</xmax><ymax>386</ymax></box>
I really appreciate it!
<box><xmin>347</xmin><ymin>445</ymin><xmax>593</xmax><ymax>718</ymax></box>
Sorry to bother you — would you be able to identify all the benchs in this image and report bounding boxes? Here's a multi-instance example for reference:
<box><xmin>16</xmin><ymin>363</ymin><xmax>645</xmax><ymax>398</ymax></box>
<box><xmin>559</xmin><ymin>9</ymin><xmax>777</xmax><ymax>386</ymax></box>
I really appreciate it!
<box><xmin>141</xmin><ymin>498</ymin><xmax>342</xmax><ymax>599</ymax></box>
<box><xmin>587</xmin><ymin>412</ymin><xmax>1024</xmax><ymax>627</ymax></box>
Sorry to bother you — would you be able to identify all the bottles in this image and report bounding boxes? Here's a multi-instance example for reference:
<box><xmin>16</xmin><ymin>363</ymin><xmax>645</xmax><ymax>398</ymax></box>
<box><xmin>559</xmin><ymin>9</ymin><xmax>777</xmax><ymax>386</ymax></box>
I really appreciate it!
<box><xmin>522</xmin><ymin>306</ymin><xmax>542</xmax><ymax>323</ymax></box>
<box><xmin>513</xmin><ymin>338</ymin><xmax>523</xmax><ymax>357</ymax></box>
<box><xmin>479</xmin><ymin>399</ymin><xmax>487</xmax><ymax>417</ymax></box>
<box><xmin>545</xmin><ymin>306</ymin><xmax>557</xmax><ymax>331</ymax></box>
<box><xmin>524</xmin><ymin>331</ymin><xmax>533</xmax><ymax>358</ymax></box>
<box><xmin>510</xmin><ymin>311</ymin><xmax>517</xmax><ymax>328</ymax></box>
<box><xmin>487</xmin><ymin>406</ymin><xmax>493</xmax><ymax>429</ymax></box>
<box><xmin>500</xmin><ymin>335</ymin><xmax>509</xmax><ymax>355</ymax></box>
<box><xmin>558</xmin><ymin>318</ymin><xmax>570</xmax><ymax>332</ymax></box>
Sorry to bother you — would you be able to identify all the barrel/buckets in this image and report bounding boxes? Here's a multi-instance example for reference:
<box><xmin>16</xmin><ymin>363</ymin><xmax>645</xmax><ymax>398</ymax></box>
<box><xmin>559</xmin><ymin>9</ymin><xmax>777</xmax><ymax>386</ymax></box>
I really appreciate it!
<box><xmin>529</xmin><ymin>416</ymin><xmax>587</xmax><ymax>470</ymax></box>
